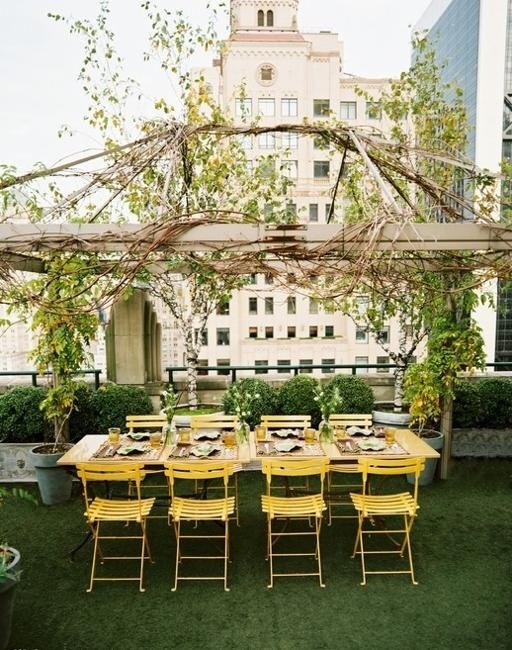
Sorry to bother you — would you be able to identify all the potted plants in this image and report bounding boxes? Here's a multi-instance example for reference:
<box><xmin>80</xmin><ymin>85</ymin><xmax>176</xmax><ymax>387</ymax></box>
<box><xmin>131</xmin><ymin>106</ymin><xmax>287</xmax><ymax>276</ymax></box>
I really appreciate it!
<box><xmin>402</xmin><ymin>314</ymin><xmax>488</xmax><ymax>489</ymax></box>
<box><xmin>29</xmin><ymin>258</ymin><xmax>98</xmax><ymax>506</ymax></box>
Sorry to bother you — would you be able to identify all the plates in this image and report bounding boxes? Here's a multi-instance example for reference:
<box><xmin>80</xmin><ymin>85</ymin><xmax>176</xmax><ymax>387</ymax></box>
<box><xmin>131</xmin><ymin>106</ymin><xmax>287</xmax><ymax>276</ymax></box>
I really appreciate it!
<box><xmin>116</xmin><ymin>447</ymin><xmax>145</xmax><ymax>455</ymax></box>
<box><xmin>194</xmin><ymin>431</ymin><xmax>221</xmax><ymax>441</ymax></box>
<box><xmin>359</xmin><ymin>440</ymin><xmax>386</xmax><ymax>451</ymax></box>
<box><xmin>274</xmin><ymin>442</ymin><xmax>303</xmax><ymax>452</ymax></box>
<box><xmin>127</xmin><ymin>432</ymin><xmax>150</xmax><ymax>440</ymax></box>
<box><xmin>190</xmin><ymin>444</ymin><xmax>221</xmax><ymax>456</ymax></box>
<box><xmin>347</xmin><ymin>426</ymin><xmax>374</xmax><ymax>436</ymax></box>
<box><xmin>271</xmin><ymin>429</ymin><xmax>299</xmax><ymax>438</ymax></box>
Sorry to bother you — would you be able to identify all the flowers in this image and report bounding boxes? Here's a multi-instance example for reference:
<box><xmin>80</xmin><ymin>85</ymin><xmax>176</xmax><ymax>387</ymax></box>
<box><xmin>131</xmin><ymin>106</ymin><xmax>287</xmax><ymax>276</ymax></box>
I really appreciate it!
<box><xmin>312</xmin><ymin>384</ymin><xmax>343</xmax><ymax>440</ymax></box>
<box><xmin>221</xmin><ymin>377</ymin><xmax>260</xmax><ymax>440</ymax></box>
<box><xmin>158</xmin><ymin>382</ymin><xmax>180</xmax><ymax>440</ymax></box>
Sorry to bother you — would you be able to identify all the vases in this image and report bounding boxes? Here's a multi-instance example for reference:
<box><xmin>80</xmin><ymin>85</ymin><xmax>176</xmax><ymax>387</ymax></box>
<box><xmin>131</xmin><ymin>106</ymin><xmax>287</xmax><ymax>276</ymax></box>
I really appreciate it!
<box><xmin>162</xmin><ymin>417</ymin><xmax>177</xmax><ymax>447</ymax></box>
<box><xmin>235</xmin><ymin>418</ymin><xmax>250</xmax><ymax>448</ymax></box>
<box><xmin>318</xmin><ymin>415</ymin><xmax>334</xmax><ymax>446</ymax></box>
<box><xmin>0</xmin><ymin>544</ymin><xmax>24</xmax><ymax>650</ymax></box>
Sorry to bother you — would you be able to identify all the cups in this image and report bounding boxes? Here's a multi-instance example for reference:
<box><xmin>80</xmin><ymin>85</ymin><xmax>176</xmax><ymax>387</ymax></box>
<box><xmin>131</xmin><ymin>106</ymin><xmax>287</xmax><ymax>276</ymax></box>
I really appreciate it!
<box><xmin>255</xmin><ymin>425</ymin><xmax>267</xmax><ymax>441</ymax></box>
<box><xmin>150</xmin><ymin>433</ymin><xmax>161</xmax><ymax>449</ymax></box>
<box><xmin>179</xmin><ymin>427</ymin><xmax>191</xmax><ymax>442</ymax></box>
<box><xmin>335</xmin><ymin>424</ymin><xmax>346</xmax><ymax>438</ymax></box>
<box><xmin>108</xmin><ymin>427</ymin><xmax>120</xmax><ymax>445</ymax></box>
<box><xmin>304</xmin><ymin>429</ymin><xmax>316</xmax><ymax>444</ymax></box>
<box><xmin>223</xmin><ymin>432</ymin><xmax>236</xmax><ymax>448</ymax></box>
<box><xmin>384</xmin><ymin>428</ymin><xmax>396</xmax><ymax>444</ymax></box>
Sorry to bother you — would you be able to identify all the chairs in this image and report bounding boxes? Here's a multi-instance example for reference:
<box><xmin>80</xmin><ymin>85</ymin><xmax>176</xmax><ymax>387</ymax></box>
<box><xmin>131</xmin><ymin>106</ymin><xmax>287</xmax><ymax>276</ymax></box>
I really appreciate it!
<box><xmin>162</xmin><ymin>459</ymin><xmax>236</xmax><ymax>593</ymax></box>
<box><xmin>190</xmin><ymin>415</ymin><xmax>241</xmax><ymax>528</ymax></box>
<box><xmin>320</xmin><ymin>413</ymin><xmax>376</xmax><ymax>528</ymax></box>
<box><xmin>124</xmin><ymin>415</ymin><xmax>173</xmax><ymax>528</ymax></box>
<box><xmin>260</xmin><ymin>457</ymin><xmax>331</xmax><ymax>590</ymax></box>
<box><xmin>75</xmin><ymin>463</ymin><xmax>157</xmax><ymax>593</ymax></box>
<box><xmin>348</xmin><ymin>456</ymin><xmax>426</xmax><ymax>586</ymax></box>
<box><xmin>260</xmin><ymin>415</ymin><xmax>315</xmax><ymax>529</ymax></box>
